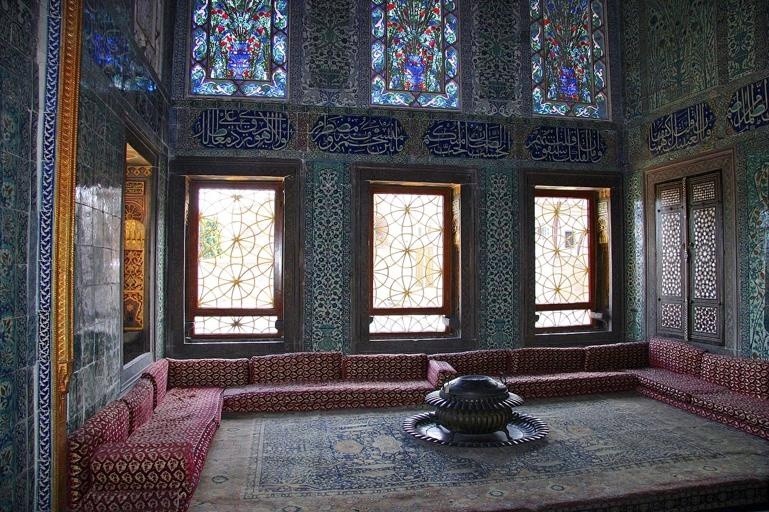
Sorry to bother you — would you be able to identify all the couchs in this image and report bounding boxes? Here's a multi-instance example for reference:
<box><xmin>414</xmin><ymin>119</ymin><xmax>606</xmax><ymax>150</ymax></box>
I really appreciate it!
<box><xmin>632</xmin><ymin>335</ymin><xmax>768</xmax><ymax>440</ymax></box>
<box><xmin>222</xmin><ymin>342</ymin><xmax>632</xmax><ymax>416</ymax></box>
<box><xmin>66</xmin><ymin>357</ymin><xmax>223</xmax><ymax>512</ymax></box>
